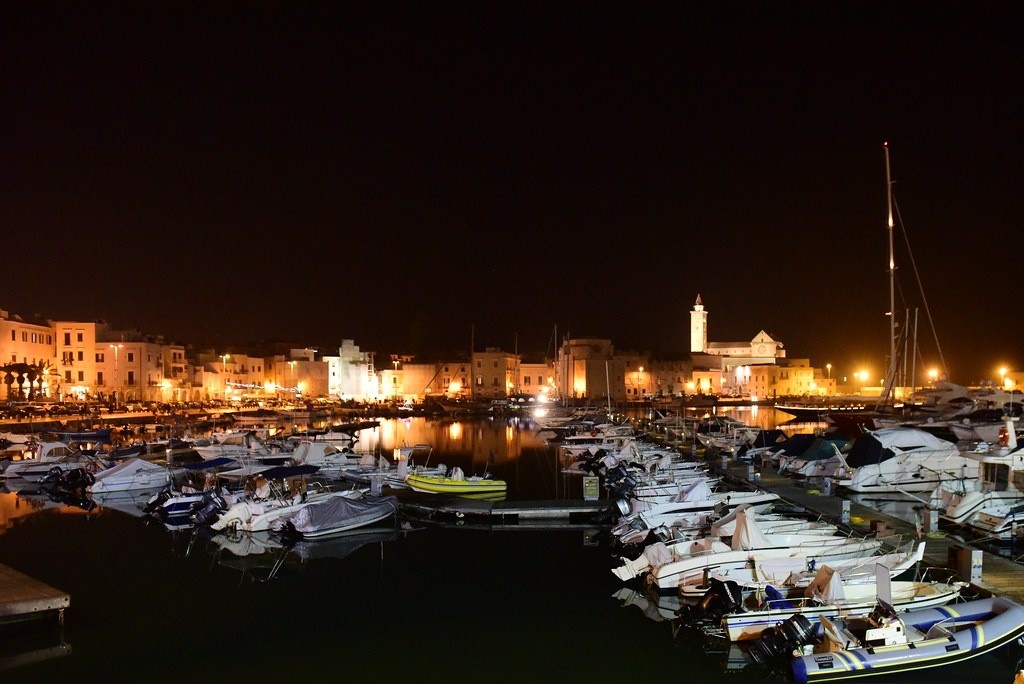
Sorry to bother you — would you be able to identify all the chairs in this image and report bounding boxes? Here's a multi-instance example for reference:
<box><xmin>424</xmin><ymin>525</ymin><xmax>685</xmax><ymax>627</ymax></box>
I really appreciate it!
<box><xmin>819</xmin><ymin>614</ymin><xmax>863</xmax><ymax>651</ymax></box>
<box><xmin>764</xmin><ymin>585</ymin><xmax>793</xmax><ymax>609</ymax></box>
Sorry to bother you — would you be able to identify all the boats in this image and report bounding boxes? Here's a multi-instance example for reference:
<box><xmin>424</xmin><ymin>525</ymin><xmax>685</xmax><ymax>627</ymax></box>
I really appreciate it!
<box><xmin>637</xmin><ymin>405</ymin><xmax>1023</xmax><ymax>545</ymax></box>
<box><xmin>538</xmin><ymin>405</ymin><xmax>964</xmax><ymax>644</ymax></box>
<box><xmin>1</xmin><ymin>426</ymin><xmax>448</xmax><ymax>539</ymax></box>
<box><xmin>746</xmin><ymin>563</ymin><xmax>1024</xmax><ymax>684</ymax></box>
<box><xmin>405</xmin><ymin>448</ymin><xmax>508</xmax><ymax>493</ymax></box>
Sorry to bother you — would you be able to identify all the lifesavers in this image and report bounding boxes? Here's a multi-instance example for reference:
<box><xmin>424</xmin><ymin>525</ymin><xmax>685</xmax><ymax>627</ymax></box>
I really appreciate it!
<box><xmin>998</xmin><ymin>427</ymin><xmax>1010</xmax><ymax>444</ymax></box>
<box><xmin>85</xmin><ymin>463</ymin><xmax>97</xmax><ymax>473</ymax></box>
<box><xmin>590</xmin><ymin>430</ymin><xmax>597</xmax><ymax>437</ymax></box>
